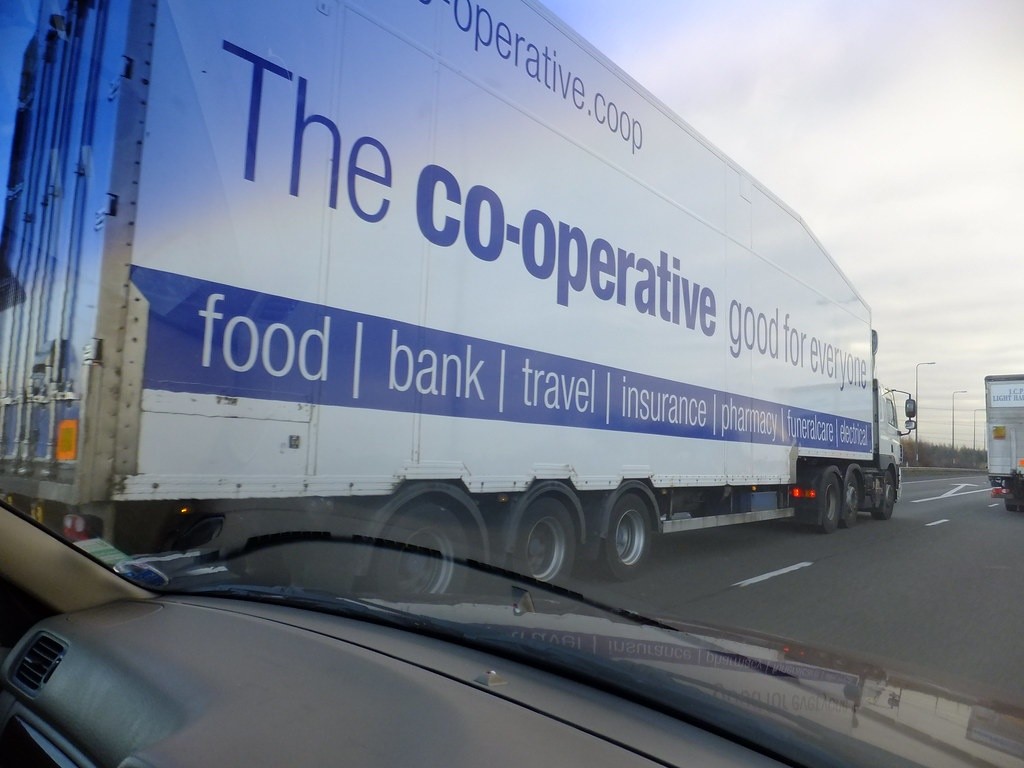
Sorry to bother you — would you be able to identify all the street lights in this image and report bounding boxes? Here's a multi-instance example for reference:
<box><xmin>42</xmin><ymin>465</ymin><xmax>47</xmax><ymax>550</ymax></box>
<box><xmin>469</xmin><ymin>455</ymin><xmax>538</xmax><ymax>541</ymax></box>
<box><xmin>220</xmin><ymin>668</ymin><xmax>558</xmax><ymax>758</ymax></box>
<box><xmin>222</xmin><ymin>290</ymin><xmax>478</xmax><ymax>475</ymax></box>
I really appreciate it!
<box><xmin>951</xmin><ymin>391</ymin><xmax>968</xmax><ymax>449</ymax></box>
<box><xmin>915</xmin><ymin>361</ymin><xmax>936</xmax><ymax>466</ymax></box>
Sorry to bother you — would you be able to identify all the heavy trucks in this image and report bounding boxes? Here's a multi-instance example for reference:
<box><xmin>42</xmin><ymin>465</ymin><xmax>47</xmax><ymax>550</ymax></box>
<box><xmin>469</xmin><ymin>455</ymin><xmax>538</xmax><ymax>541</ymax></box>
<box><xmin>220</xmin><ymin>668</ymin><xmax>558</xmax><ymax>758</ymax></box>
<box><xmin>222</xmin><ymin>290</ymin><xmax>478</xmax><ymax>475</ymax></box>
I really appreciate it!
<box><xmin>1</xmin><ymin>1</ymin><xmax>920</xmax><ymax>626</ymax></box>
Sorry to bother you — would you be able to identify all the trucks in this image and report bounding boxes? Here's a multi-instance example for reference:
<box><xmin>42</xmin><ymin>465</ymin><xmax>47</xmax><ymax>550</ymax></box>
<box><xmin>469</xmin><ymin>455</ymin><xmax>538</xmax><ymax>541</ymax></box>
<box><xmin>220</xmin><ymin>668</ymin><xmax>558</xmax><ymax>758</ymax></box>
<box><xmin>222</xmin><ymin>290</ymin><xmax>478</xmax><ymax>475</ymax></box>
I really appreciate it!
<box><xmin>984</xmin><ymin>373</ymin><xmax>1024</xmax><ymax>513</ymax></box>
